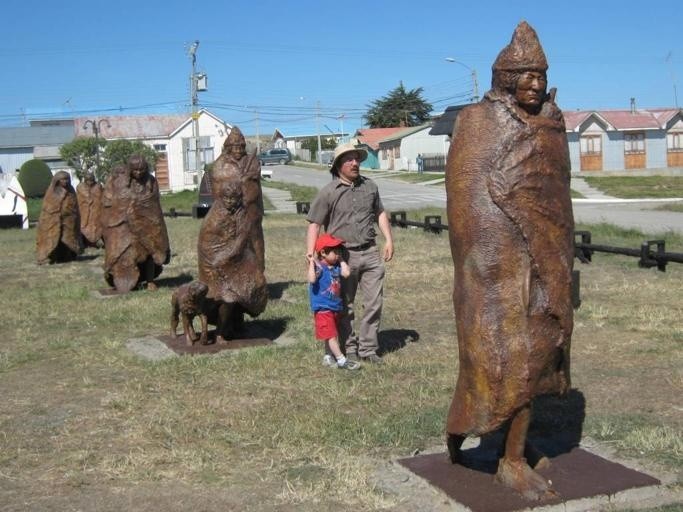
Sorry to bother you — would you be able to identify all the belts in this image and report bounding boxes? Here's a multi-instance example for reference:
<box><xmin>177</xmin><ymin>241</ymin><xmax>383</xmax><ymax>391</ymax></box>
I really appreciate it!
<box><xmin>346</xmin><ymin>239</ymin><xmax>376</xmax><ymax>251</ymax></box>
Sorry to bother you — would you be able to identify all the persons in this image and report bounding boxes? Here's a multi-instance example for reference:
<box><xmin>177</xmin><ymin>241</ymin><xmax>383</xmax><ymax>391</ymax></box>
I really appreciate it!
<box><xmin>104</xmin><ymin>155</ymin><xmax>170</xmax><ymax>293</ymax></box>
<box><xmin>305</xmin><ymin>142</ymin><xmax>394</xmax><ymax>366</ymax></box>
<box><xmin>75</xmin><ymin>173</ymin><xmax>104</xmax><ymax>248</ymax></box>
<box><xmin>416</xmin><ymin>154</ymin><xmax>423</xmax><ymax>175</ymax></box>
<box><xmin>104</xmin><ymin>166</ymin><xmax>125</xmax><ymax>205</ymax></box>
<box><xmin>305</xmin><ymin>232</ymin><xmax>362</xmax><ymax>371</ymax></box>
<box><xmin>197</xmin><ymin>180</ymin><xmax>267</xmax><ymax>336</ymax></box>
<box><xmin>443</xmin><ymin>21</ymin><xmax>576</xmax><ymax>502</ymax></box>
<box><xmin>35</xmin><ymin>170</ymin><xmax>81</xmax><ymax>266</ymax></box>
<box><xmin>210</xmin><ymin>127</ymin><xmax>265</xmax><ymax>273</ymax></box>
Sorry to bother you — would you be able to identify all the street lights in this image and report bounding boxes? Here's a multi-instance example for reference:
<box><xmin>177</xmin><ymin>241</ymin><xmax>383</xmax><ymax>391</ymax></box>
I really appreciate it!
<box><xmin>244</xmin><ymin>105</ymin><xmax>260</xmax><ymax>154</ymax></box>
<box><xmin>444</xmin><ymin>58</ymin><xmax>479</xmax><ymax>104</ymax></box>
<box><xmin>299</xmin><ymin>96</ymin><xmax>322</xmax><ymax>168</ymax></box>
<box><xmin>82</xmin><ymin>119</ymin><xmax>111</xmax><ymax>184</ymax></box>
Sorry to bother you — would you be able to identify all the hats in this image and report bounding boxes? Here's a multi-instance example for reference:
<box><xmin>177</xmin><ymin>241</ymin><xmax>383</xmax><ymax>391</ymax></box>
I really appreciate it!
<box><xmin>315</xmin><ymin>233</ymin><xmax>346</xmax><ymax>253</ymax></box>
<box><xmin>329</xmin><ymin>142</ymin><xmax>368</xmax><ymax>177</ymax></box>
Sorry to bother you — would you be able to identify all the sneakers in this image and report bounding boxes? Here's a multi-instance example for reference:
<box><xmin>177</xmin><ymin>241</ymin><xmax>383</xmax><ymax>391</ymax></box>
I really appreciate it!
<box><xmin>322</xmin><ymin>355</ymin><xmax>337</xmax><ymax>366</ymax></box>
<box><xmin>338</xmin><ymin>358</ymin><xmax>361</xmax><ymax>370</ymax></box>
<box><xmin>359</xmin><ymin>354</ymin><xmax>382</xmax><ymax>364</ymax></box>
<box><xmin>346</xmin><ymin>353</ymin><xmax>358</xmax><ymax>362</ymax></box>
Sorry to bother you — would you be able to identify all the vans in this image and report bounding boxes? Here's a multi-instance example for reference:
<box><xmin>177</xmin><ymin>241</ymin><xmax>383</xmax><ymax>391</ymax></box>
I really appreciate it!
<box><xmin>256</xmin><ymin>149</ymin><xmax>291</xmax><ymax>166</ymax></box>
